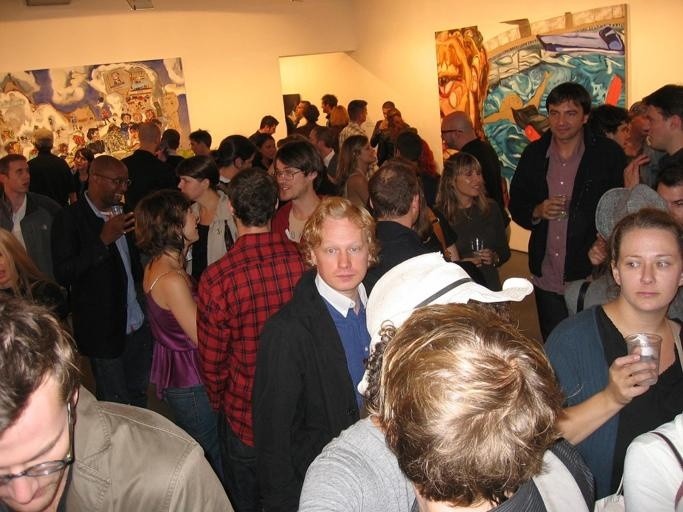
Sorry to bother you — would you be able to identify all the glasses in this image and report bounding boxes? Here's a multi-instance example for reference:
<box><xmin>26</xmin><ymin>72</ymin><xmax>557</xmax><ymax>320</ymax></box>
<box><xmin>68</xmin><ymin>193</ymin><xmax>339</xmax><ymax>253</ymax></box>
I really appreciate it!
<box><xmin>273</xmin><ymin>169</ymin><xmax>302</xmax><ymax>179</ymax></box>
<box><xmin>1</xmin><ymin>403</ymin><xmax>75</xmax><ymax>485</ymax></box>
<box><xmin>98</xmin><ymin>173</ymin><xmax>131</xmax><ymax>187</ymax></box>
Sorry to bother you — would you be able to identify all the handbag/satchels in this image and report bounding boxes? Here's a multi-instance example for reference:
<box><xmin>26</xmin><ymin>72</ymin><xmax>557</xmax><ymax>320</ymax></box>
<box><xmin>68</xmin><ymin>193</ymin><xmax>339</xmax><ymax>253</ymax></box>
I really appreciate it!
<box><xmin>594</xmin><ymin>494</ymin><xmax>626</xmax><ymax>512</ymax></box>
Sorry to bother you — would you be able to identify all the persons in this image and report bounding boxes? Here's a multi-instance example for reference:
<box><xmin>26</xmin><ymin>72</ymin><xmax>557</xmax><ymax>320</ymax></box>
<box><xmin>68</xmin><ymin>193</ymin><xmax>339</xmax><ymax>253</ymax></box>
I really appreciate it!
<box><xmin>0</xmin><ymin>82</ymin><xmax>682</xmax><ymax>512</ymax></box>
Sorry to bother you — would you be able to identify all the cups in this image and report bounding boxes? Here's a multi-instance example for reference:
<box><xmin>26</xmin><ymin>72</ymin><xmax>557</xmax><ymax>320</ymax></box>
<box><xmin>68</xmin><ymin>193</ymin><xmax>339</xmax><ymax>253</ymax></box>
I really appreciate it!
<box><xmin>625</xmin><ymin>332</ymin><xmax>660</xmax><ymax>386</ymax></box>
<box><xmin>471</xmin><ymin>239</ymin><xmax>484</xmax><ymax>268</ymax></box>
<box><xmin>111</xmin><ymin>205</ymin><xmax>124</xmax><ymax>216</ymax></box>
<box><xmin>554</xmin><ymin>195</ymin><xmax>570</xmax><ymax>220</ymax></box>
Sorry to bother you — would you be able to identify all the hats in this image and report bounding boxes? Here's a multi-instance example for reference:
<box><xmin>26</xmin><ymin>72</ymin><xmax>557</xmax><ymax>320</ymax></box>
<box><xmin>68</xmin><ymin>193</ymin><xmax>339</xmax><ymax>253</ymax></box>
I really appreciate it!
<box><xmin>356</xmin><ymin>250</ymin><xmax>534</xmax><ymax>397</ymax></box>
<box><xmin>594</xmin><ymin>182</ymin><xmax>667</xmax><ymax>243</ymax></box>
<box><xmin>34</xmin><ymin>130</ymin><xmax>54</xmax><ymax>148</ymax></box>
